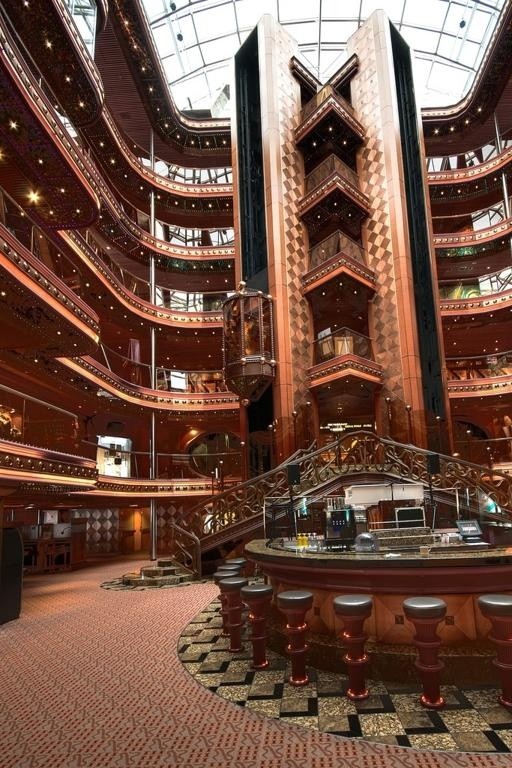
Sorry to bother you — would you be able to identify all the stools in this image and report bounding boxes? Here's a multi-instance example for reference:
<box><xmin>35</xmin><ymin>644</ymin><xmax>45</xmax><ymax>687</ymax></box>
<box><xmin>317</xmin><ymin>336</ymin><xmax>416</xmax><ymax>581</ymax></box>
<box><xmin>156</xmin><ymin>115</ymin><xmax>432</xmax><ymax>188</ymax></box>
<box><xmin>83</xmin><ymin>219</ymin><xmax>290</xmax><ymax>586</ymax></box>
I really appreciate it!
<box><xmin>276</xmin><ymin>589</ymin><xmax>315</xmax><ymax>687</ymax></box>
<box><xmin>332</xmin><ymin>593</ymin><xmax>372</xmax><ymax>701</ymax></box>
<box><xmin>402</xmin><ymin>595</ymin><xmax>448</xmax><ymax>709</ymax></box>
<box><xmin>240</xmin><ymin>583</ymin><xmax>274</xmax><ymax>668</ymax></box>
<box><xmin>214</xmin><ymin>557</ymin><xmax>249</xmax><ymax>654</ymax></box>
<box><xmin>477</xmin><ymin>594</ymin><xmax>511</xmax><ymax>709</ymax></box>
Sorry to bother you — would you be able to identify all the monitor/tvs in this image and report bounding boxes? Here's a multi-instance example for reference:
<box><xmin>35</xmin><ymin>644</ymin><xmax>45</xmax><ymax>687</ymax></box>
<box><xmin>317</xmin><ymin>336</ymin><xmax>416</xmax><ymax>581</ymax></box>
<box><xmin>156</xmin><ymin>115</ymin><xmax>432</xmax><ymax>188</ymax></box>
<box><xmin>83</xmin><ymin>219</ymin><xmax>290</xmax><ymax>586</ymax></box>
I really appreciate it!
<box><xmin>456</xmin><ymin>518</ymin><xmax>484</xmax><ymax>538</ymax></box>
<box><xmin>394</xmin><ymin>505</ymin><xmax>427</xmax><ymax>528</ymax></box>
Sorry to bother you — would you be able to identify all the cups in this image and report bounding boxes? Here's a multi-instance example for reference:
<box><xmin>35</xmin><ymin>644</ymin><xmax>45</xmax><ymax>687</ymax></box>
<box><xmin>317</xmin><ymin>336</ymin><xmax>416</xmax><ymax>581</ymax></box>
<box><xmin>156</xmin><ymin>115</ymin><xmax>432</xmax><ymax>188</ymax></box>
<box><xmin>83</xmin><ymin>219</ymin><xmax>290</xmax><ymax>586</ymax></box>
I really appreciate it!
<box><xmin>419</xmin><ymin>545</ymin><xmax>432</xmax><ymax>558</ymax></box>
